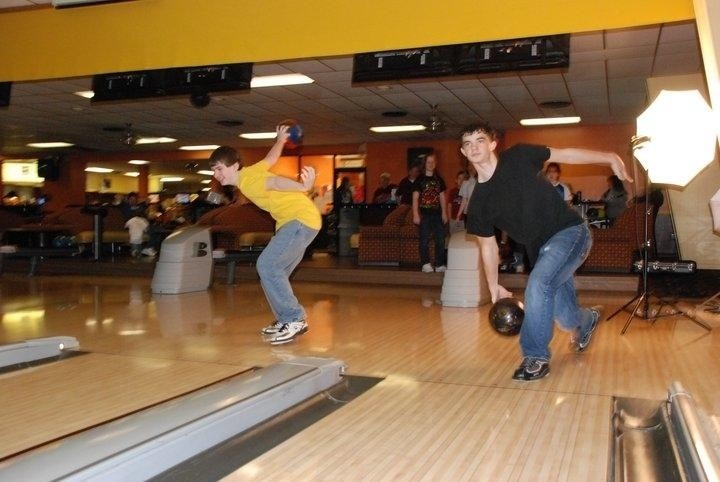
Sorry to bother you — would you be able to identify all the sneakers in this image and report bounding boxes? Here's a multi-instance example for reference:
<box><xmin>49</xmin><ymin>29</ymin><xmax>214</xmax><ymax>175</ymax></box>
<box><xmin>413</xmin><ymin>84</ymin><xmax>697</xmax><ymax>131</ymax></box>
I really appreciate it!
<box><xmin>568</xmin><ymin>306</ymin><xmax>601</xmax><ymax>354</ymax></box>
<box><xmin>260</xmin><ymin>320</ymin><xmax>311</xmax><ymax>345</ymax></box>
<box><xmin>140</xmin><ymin>247</ymin><xmax>156</xmax><ymax>256</ymax></box>
<box><xmin>511</xmin><ymin>358</ymin><xmax>550</xmax><ymax>382</ymax></box>
<box><xmin>421</xmin><ymin>263</ymin><xmax>434</xmax><ymax>273</ymax></box>
<box><xmin>436</xmin><ymin>265</ymin><xmax>446</xmax><ymax>272</ymax></box>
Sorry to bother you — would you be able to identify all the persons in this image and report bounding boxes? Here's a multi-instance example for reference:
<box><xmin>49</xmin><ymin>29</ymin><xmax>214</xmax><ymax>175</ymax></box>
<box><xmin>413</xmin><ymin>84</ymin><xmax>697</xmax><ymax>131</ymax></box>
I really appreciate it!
<box><xmin>444</xmin><ymin>169</ymin><xmax>470</xmax><ymax>235</ymax></box>
<box><xmin>116</xmin><ymin>179</ymin><xmax>233</xmax><ymax>256</ymax></box>
<box><xmin>545</xmin><ymin>161</ymin><xmax>573</xmax><ymax>207</ymax></box>
<box><xmin>372</xmin><ymin>172</ymin><xmax>398</xmax><ymax>226</ymax></box>
<box><xmin>334</xmin><ymin>178</ymin><xmax>353</xmax><ymax>254</ymax></box>
<box><xmin>453</xmin><ymin>161</ymin><xmax>478</xmax><ymax>231</ymax></box>
<box><xmin>395</xmin><ymin>159</ymin><xmax>421</xmax><ymax>206</ymax></box>
<box><xmin>599</xmin><ymin>174</ymin><xmax>629</xmax><ymax>219</ymax></box>
<box><xmin>458</xmin><ymin>118</ymin><xmax>635</xmax><ymax>385</ymax></box>
<box><xmin>412</xmin><ymin>152</ymin><xmax>450</xmax><ymax>275</ymax></box>
<box><xmin>209</xmin><ymin>124</ymin><xmax>326</xmax><ymax>345</ymax></box>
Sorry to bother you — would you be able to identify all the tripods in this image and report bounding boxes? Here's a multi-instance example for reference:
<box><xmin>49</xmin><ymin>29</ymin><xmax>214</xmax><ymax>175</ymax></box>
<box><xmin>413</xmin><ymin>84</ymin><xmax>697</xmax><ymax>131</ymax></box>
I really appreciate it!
<box><xmin>606</xmin><ymin>170</ymin><xmax>712</xmax><ymax>335</ymax></box>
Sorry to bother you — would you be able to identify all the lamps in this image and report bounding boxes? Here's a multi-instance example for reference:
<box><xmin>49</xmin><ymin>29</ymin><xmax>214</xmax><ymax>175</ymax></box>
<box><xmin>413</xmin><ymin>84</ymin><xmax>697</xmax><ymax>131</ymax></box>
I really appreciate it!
<box><xmin>606</xmin><ymin>89</ymin><xmax>716</xmax><ymax>338</ymax></box>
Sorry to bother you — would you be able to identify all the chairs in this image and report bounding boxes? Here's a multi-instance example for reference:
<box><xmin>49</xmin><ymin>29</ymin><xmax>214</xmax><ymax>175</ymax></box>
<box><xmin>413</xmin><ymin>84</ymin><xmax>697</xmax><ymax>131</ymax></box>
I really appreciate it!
<box><xmin>401</xmin><ymin>209</ymin><xmax>436</xmax><ymax>268</ymax></box>
<box><xmin>0</xmin><ymin>203</ymin><xmax>276</xmax><ymax>266</ymax></box>
<box><xmin>359</xmin><ymin>204</ymin><xmax>411</xmax><ymax>266</ymax></box>
<box><xmin>576</xmin><ymin>202</ymin><xmax>654</xmax><ymax>273</ymax></box>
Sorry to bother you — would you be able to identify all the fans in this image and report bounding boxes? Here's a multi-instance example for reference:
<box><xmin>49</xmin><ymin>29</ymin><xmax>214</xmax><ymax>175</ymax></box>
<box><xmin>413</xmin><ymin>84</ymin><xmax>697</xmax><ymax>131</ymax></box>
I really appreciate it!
<box><xmin>424</xmin><ymin>104</ymin><xmax>465</xmax><ymax>135</ymax></box>
<box><xmin>105</xmin><ymin>122</ymin><xmax>138</xmax><ymax>150</ymax></box>
